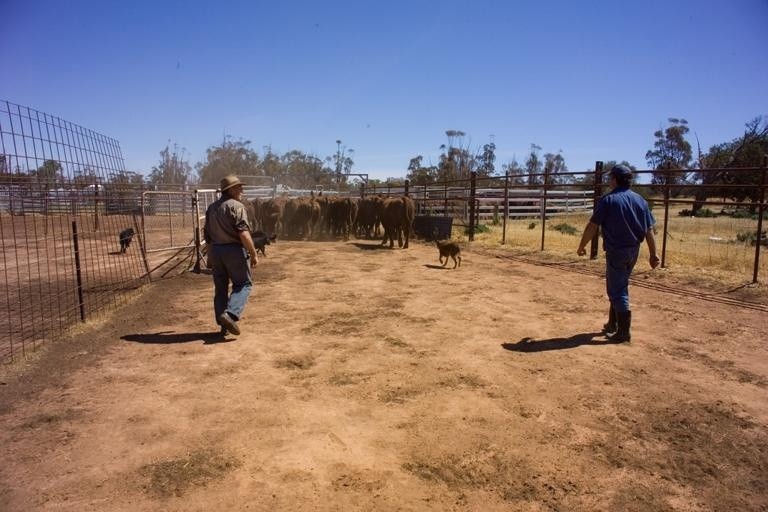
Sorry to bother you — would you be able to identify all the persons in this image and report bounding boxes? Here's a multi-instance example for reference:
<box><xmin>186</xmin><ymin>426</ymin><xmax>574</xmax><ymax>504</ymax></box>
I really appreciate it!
<box><xmin>576</xmin><ymin>163</ymin><xmax>662</xmax><ymax>343</ymax></box>
<box><xmin>204</xmin><ymin>175</ymin><xmax>258</xmax><ymax>343</ymax></box>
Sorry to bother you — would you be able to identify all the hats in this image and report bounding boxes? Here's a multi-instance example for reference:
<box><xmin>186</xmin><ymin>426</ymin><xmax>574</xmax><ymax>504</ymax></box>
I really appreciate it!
<box><xmin>216</xmin><ymin>175</ymin><xmax>246</xmax><ymax>192</ymax></box>
<box><xmin>605</xmin><ymin>164</ymin><xmax>632</xmax><ymax>177</ymax></box>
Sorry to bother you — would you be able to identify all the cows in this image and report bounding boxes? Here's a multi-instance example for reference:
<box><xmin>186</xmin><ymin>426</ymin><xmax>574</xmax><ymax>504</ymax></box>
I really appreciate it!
<box><xmin>241</xmin><ymin>190</ymin><xmax>416</xmax><ymax>249</ymax></box>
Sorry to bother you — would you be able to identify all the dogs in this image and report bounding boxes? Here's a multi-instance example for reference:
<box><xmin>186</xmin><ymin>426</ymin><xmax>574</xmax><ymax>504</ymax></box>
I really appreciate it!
<box><xmin>252</xmin><ymin>236</ymin><xmax>270</xmax><ymax>257</ymax></box>
<box><xmin>119</xmin><ymin>228</ymin><xmax>135</xmax><ymax>253</ymax></box>
<box><xmin>250</xmin><ymin>231</ymin><xmax>277</xmax><ymax>254</ymax></box>
<box><xmin>435</xmin><ymin>239</ymin><xmax>461</xmax><ymax>269</ymax></box>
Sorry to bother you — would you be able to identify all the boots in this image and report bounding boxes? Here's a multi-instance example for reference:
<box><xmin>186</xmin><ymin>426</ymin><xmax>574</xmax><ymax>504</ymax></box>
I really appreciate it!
<box><xmin>603</xmin><ymin>303</ymin><xmax>631</xmax><ymax>343</ymax></box>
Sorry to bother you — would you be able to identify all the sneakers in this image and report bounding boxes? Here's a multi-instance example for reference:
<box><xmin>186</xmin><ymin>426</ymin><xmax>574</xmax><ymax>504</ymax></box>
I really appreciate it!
<box><xmin>219</xmin><ymin>312</ymin><xmax>241</xmax><ymax>336</ymax></box>
